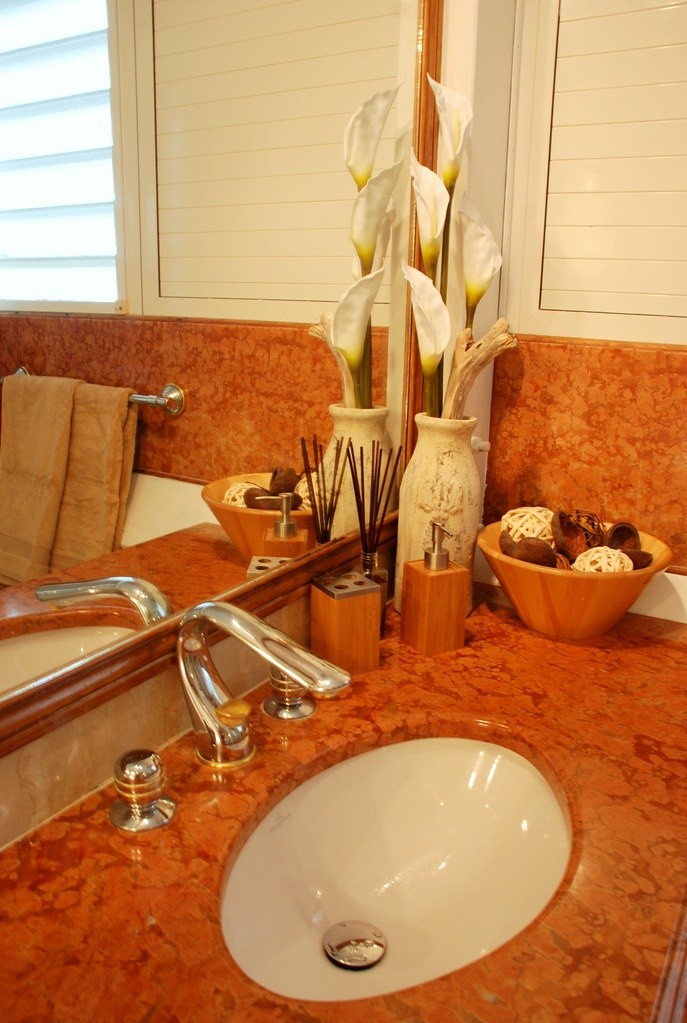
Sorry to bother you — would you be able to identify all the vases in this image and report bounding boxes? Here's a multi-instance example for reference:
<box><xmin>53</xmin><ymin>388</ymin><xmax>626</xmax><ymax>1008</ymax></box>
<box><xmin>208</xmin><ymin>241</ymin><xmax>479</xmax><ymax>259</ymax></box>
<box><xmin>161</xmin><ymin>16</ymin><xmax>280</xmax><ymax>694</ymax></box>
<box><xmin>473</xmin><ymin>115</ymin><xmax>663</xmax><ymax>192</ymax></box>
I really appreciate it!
<box><xmin>391</xmin><ymin>415</ymin><xmax>484</xmax><ymax>616</ymax></box>
<box><xmin>309</xmin><ymin>406</ymin><xmax>399</xmax><ymax>540</ymax></box>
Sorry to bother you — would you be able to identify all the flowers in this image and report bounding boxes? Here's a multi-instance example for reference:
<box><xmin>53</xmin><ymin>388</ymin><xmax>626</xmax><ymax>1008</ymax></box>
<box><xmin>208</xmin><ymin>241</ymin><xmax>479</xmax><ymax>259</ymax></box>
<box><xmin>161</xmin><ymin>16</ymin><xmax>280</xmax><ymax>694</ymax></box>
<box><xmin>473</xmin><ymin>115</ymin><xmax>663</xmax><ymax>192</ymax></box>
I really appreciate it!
<box><xmin>398</xmin><ymin>73</ymin><xmax>503</xmax><ymax>417</ymax></box>
<box><xmin>306</xmin><ymin>88</ymin><xmax>410</xmax><ymax>412</ymax></box>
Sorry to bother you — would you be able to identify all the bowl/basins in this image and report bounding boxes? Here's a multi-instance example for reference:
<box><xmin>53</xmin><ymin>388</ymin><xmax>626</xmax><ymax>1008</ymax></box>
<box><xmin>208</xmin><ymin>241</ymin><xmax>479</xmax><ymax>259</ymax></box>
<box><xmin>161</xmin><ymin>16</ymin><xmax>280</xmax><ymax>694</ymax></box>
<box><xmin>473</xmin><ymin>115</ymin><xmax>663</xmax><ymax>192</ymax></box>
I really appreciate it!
<box><xmin>476</xmin><ymin>520</ymin><xmax>672</xmax><ymax>642</ymax></box>
<box><xmin>202</xmin><ymin>470</ymin><xmax>301</xmax><ymax>564</ymax></box>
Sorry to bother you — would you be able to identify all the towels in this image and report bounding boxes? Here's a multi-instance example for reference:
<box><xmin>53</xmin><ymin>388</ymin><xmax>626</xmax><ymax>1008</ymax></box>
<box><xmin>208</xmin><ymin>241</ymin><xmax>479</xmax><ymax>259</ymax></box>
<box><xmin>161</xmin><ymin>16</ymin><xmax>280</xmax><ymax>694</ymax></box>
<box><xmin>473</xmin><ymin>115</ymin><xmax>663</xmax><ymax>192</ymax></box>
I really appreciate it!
<box><xmin>0</xmin><ymin>375</ymin><xmax>139</xmax><ymax>586</ymax></box>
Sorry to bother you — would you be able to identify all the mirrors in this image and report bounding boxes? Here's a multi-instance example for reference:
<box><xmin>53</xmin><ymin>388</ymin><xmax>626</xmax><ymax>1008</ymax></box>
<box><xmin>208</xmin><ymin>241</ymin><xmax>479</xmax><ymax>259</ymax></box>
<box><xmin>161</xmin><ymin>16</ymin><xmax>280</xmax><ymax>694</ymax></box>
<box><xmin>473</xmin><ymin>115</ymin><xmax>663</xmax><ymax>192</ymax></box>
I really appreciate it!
<box><xmin>0</xmin><ymin>0</ymin><xmax>445</xmax><ymax>756</ymax></box>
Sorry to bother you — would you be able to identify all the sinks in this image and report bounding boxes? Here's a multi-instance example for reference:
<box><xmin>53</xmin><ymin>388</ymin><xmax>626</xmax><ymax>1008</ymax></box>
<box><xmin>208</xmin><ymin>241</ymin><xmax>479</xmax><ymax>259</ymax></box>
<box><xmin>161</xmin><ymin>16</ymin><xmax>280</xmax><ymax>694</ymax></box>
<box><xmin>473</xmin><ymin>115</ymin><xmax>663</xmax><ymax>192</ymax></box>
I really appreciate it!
<box><xmin>0</xmin><ymin>606</ymin><xmax>145</xmax><ymax>693</ymax></box>
<box><xmin>213</xmin><ymin>713</ymin><xmax>584</xmax><ymax>1011</ymax></box>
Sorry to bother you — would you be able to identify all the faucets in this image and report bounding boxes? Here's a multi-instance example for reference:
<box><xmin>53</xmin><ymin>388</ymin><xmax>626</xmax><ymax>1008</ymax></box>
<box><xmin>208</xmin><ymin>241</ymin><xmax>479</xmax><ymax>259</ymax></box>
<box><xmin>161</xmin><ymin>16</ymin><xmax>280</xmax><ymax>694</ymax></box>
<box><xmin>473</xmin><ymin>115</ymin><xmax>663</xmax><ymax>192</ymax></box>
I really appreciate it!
<box><xmin>34</xmin><ymin>576</ymin><xmax>171</xmax><ymax>626</ymax></box>
<box><xmin>178</xmin><ymin>601</ymin><xmax>351</xmax><ymax>767</ymax></box>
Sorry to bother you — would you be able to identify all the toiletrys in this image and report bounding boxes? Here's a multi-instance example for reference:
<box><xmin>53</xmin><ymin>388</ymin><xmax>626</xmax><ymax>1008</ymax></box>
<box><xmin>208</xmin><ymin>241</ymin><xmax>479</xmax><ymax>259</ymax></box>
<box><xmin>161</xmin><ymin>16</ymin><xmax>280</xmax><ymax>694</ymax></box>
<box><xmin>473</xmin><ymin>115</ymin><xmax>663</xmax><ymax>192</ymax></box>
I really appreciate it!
<box><xmin>400</xmin><ymin>521</ymin><xmax>469</xmax><ymax>656</ymax></box>
<box><xmin>255</xmin><ymin>494</ymin><xmax>308</xmax><ymax>557</ymax></box>
